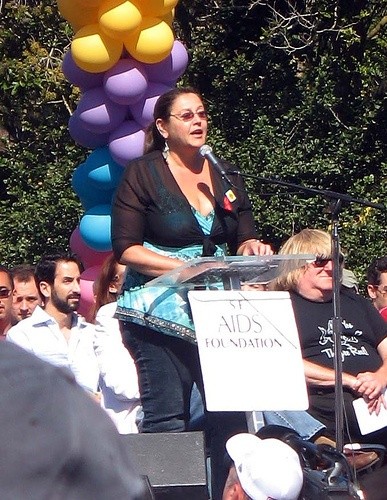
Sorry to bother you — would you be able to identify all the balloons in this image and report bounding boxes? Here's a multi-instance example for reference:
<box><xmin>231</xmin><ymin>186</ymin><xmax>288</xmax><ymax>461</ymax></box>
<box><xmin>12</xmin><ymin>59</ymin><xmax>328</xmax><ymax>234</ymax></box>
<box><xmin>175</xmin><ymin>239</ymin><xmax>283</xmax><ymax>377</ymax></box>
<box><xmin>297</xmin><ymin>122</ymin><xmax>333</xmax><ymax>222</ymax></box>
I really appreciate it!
<box><xmin>56</xmin><ymin>0</ymin><xmax>189</xmax><ymax>323</ymax></box>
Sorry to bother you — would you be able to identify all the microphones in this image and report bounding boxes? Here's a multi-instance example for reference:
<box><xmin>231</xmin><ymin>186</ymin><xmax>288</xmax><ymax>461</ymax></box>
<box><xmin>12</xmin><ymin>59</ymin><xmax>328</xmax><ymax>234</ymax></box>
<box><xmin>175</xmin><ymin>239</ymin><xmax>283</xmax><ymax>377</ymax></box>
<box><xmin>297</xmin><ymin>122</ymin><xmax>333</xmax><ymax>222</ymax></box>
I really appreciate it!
<box><xmin>199</xmin><ymin>144</ymin><xmax>231</xmax><ymax>182</ymax></box>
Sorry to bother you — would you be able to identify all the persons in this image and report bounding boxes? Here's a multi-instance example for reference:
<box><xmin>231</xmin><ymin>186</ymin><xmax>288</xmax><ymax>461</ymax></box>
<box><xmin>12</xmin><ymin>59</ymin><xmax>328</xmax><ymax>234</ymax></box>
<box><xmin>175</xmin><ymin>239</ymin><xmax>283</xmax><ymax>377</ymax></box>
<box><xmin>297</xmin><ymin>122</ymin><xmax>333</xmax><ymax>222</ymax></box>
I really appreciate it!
<box><xmin>5</xmin><ymin>246</ymin><xmax>101</xmax><ymax>403</ymax></box>
<box><xmin>11</xmin><ymin>262</ymin><xmax>44</xmax><ymax>327</ymax></box>
<box><xmin>110</xmin><ymin>87</ymin><xmax>273</xmax><ymax>500</ymax></box>
<box><xmin>90</xmin><ymin>252</ymin><xmax>126</xmax><ymax>320</ymax></box>
<box><xmin>222</xmin><ymin>441</ymin><xmax>302</xmax><ymax>500</ymax></box>
<box><xmin>357</xmin><ymin>465</ymin><xmax>387</xmax><ymax>500</ymax></box>
<box><xmin>0</xmin><ymin>339</ymin><xmax>149</xmax><ymax>500</ymax></box>
<box><xmin>341</xmin><ymin>268</ymin><xmax>359</xmax><ymax>295</ymax></box>
<box><xmin>268</xmin><ymin>229</ymin><xmax>387</xmax><ymax>478</ymax></box>
<box><xmin>241</xmin><ymin>284</ymin><xmax>270</xmax><ymax>292</ymax></box>
<box><xmin>94</xmin><ymin>301</ymin><xmax>380</xmax><ymax>497</ymax></box>
<box><xmin>367</xmin><ymin>257</ymin><xmax>387</xmax><ymax>323</ymax></box>
<box><xmin>0</xmin><ymin>264</ymin><xmax>14</xmax><ymax>338</ymax></box>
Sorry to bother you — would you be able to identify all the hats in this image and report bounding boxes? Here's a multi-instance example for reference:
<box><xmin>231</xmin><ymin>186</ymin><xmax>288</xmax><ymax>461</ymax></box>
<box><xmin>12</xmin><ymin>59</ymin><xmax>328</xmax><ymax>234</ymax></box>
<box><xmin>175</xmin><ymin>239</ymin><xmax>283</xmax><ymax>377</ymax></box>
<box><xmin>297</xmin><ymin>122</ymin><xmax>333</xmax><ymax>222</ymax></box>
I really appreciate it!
<box><xmin>225</xmin><ymin>432</ymin><xmax>303</xmax><ymax>500</ymax></box>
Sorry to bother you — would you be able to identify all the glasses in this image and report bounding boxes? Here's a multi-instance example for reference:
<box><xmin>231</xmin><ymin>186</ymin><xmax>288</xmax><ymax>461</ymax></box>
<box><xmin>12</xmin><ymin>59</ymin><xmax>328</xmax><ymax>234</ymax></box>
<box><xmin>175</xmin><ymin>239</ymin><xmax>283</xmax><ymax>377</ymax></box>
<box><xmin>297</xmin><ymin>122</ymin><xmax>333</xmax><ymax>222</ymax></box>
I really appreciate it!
<box><xmin>373</xmin><ymin>285</ymin><xmax>387</xmax><ymax>294</ymax></box>
<box><xmin>312</xmin><ymin>254</ymin><xmax>344</xmax><ymax>268</ymax></box>
<box><xmin>0</xmin><ymin>289</ymin><xmax>13</xmax><ymax>299</ymax></box>
<box><xmin>165</xmin><ymin>110</ymin><xmax>209</xmax><ymax>122</ymax></box>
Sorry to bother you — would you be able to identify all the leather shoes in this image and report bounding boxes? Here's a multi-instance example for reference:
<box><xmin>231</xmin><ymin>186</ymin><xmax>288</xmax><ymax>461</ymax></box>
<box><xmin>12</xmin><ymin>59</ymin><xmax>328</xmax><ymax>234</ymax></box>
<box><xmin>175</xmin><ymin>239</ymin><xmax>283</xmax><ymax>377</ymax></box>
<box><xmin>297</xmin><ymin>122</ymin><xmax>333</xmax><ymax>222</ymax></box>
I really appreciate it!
<box><xmin>343</xmin><ymin>448</ymin><xmax>380</xmax><ymax>472</ymax></box>
